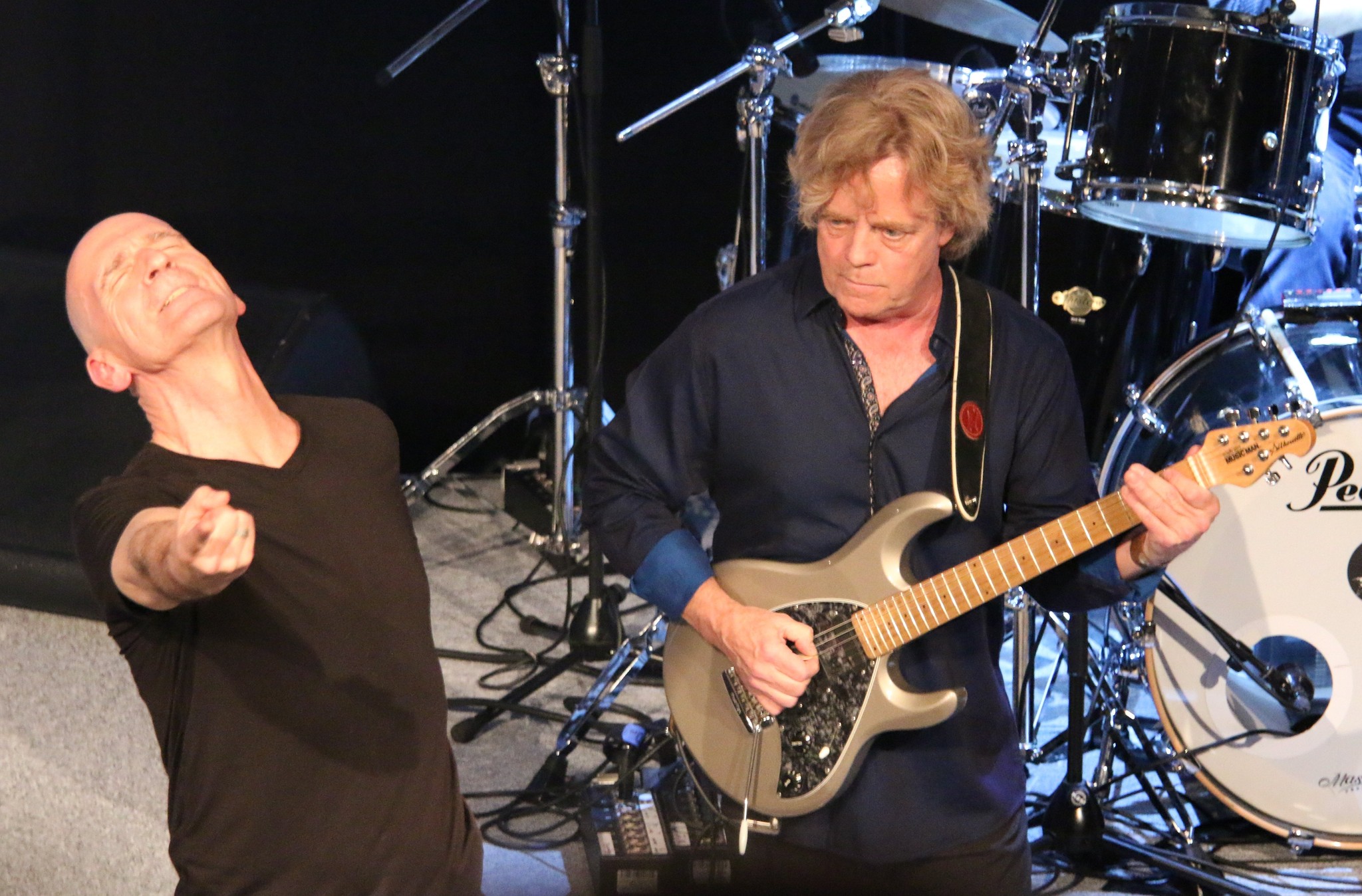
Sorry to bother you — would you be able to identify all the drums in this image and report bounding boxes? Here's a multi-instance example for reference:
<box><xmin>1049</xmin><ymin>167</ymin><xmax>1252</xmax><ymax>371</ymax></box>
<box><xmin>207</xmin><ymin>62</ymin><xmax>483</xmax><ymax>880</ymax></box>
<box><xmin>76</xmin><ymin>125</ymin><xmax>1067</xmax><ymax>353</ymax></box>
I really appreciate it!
<box><xmin>720</xmin><ymin>55</ymin><xmax>1156</xmax><ymax>423</ymax></box>
<box><xmin>1077</xmin><ymin>1</ymin><xmax>1347</xmax><ymax>251</ymax></box>
<box><xmin>1097</xmin><ymin>306</ymin><xmax>1362</xmax><ymax>853</ymax></box>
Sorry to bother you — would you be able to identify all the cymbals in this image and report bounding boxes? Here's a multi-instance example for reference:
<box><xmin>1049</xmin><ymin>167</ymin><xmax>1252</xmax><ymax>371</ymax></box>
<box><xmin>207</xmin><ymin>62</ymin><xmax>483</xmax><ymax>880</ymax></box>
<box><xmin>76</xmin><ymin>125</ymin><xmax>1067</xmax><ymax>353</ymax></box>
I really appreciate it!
<box><xmin>882</xmin><ymin>0</ymin><xmax>1070</xmax><ymax>55</ymax></box>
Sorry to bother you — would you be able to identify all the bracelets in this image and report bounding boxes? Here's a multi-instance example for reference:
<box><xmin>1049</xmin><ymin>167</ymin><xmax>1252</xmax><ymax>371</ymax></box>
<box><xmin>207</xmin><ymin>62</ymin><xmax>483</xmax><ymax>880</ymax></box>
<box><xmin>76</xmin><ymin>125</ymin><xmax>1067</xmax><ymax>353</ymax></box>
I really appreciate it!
<box><xmin>1131</xmin><ymin>533</ymin><xmax>1155</xmax><ymax>569</ymax></box>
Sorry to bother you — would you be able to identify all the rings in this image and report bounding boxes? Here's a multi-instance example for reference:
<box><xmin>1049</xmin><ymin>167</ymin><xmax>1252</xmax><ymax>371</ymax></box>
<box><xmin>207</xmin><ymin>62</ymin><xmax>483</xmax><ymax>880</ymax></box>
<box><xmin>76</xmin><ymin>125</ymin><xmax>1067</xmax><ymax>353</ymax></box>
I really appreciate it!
<box><xmin>237</xmin><ymin>528</ymin><xmax>248</xmax><ymax>536</ymax></box>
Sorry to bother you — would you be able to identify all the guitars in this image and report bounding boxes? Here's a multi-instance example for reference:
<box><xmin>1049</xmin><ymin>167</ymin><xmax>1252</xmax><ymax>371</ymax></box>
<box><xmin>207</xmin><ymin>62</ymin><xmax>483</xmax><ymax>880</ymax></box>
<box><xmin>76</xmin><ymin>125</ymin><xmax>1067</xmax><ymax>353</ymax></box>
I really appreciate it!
<box><xmin>660</xmin><ymin>400</ymin><xmax>1316</xmax><ymax>821</ymax></box>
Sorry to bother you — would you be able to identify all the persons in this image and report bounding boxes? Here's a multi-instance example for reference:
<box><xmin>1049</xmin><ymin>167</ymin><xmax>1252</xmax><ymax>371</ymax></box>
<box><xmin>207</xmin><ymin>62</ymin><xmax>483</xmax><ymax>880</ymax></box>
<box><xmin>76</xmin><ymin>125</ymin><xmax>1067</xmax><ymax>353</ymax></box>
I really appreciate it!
<box><xmin>577</xmin><ymin>74</ymin><xmax>1221</xmax><ymax>896</ymax></box>
<box><xmin>65</xmin><ymin>213</ymin><xmax>482</xmax><ymax>894</ymax></box>
<box><xmin>1208</xmin><ymin>0</ymin><xmax>1362</xmax><ymax>314</ymax></box>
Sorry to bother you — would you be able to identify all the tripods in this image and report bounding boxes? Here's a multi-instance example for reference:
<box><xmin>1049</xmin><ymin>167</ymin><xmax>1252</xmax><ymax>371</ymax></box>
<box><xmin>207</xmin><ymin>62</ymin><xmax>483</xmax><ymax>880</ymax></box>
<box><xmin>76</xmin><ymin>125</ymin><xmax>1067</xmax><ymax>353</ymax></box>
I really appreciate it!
<box><xmin>383</xmin><ymin>0</ymin><xmax>666</xmax><ymax>745</ymax></box>
<box><xmin>983</xmin><ymin>1</ymin><xmax>1278</xmax><ymax>896</ymax></box>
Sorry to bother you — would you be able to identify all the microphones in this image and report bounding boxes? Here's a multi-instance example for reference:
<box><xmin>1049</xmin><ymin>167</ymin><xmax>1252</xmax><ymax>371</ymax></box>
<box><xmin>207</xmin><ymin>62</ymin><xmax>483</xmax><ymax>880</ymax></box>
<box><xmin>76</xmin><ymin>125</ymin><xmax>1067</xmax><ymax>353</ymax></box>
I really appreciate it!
<box><xmin>1261</xmin><ymin>662</ymin><xmax>1316</xmax><ymax>713</ymax></box>
